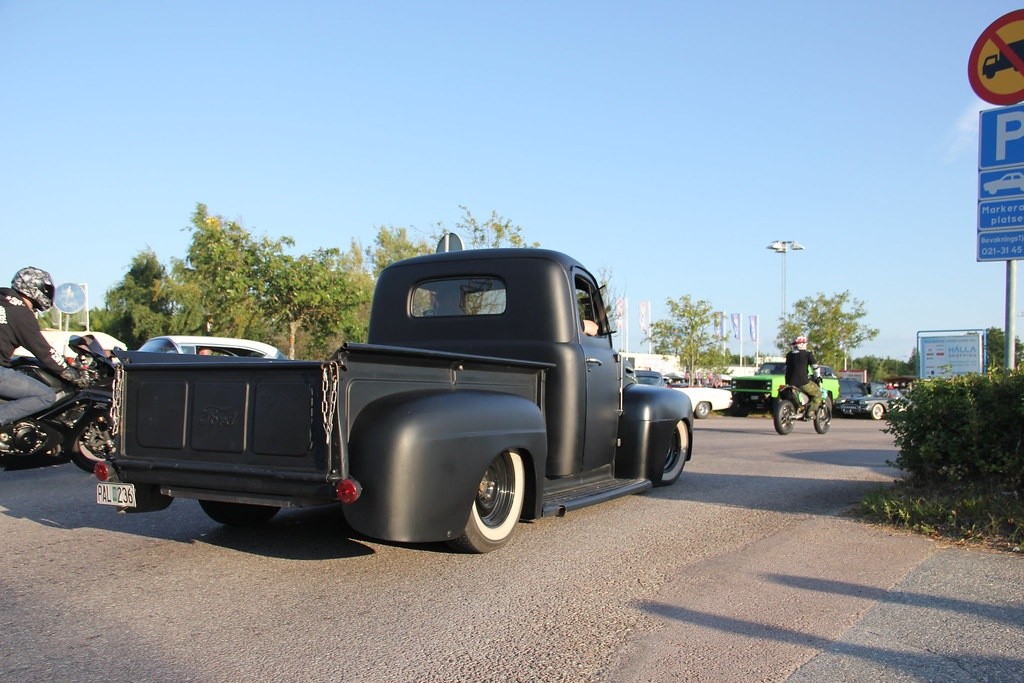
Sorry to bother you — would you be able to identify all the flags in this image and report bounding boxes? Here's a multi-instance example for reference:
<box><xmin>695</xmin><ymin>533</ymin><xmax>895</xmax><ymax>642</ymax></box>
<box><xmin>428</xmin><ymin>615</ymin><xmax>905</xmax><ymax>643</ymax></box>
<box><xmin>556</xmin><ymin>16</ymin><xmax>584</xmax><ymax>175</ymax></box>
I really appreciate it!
<box><xmin>748</xmin><ymin>315</ymin><xmax>757</xmax><ymax>341</ymax></box>
<box><xmin>615</xmin><ymin>297</ymin><xmax>627</xmax><ymax>329</ymax></box>
<box><xmin>731</xmin><ymin>314</ymin><xmax>740</xmax><ymax>340</ymax></box>
<box><xmin>713</xmin><ymin>312</ymin><xmax>723</xmax><ymax>338</ymax></box>
<box><xmin>640</xmin><ymin>303</ymin><xmax>649</xmax><ymax>332</ymax></box>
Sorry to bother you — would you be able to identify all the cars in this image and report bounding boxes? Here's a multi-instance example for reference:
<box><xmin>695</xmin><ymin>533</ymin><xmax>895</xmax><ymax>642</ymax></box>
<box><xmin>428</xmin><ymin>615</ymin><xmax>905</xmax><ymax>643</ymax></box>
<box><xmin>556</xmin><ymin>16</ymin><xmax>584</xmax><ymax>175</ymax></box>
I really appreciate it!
<box><xmin>136</xmin><ymin>335</ymin><xmax>290</xmax><ymax>361</ymax></box>
<box><xmin>835</xmin><ymin>378</ymin><xmax>915</xmax><ymax>420</ymax></box>
<box><xmin>632</xmin><ymin>370</ymin><xmax>734</xmax><ymax>419</ymax></box>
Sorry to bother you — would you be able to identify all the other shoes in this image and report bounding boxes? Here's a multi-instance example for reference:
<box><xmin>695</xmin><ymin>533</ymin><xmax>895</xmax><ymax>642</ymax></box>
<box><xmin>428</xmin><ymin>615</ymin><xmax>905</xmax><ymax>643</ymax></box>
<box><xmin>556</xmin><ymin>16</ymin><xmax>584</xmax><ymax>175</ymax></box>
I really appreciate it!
<box><xmin>806</xmin><ymin>408</ymin><xmax>816</xmax><ymax>420</ymax></box>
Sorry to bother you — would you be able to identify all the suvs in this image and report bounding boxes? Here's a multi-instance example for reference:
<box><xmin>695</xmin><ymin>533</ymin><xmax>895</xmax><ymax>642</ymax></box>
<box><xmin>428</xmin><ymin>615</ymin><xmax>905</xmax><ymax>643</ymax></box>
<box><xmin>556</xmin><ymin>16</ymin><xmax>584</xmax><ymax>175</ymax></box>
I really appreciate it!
<box><xmin>730</xmin><ymin>362</ymin><xmax>840</xmax><ymax>417</ymax></box>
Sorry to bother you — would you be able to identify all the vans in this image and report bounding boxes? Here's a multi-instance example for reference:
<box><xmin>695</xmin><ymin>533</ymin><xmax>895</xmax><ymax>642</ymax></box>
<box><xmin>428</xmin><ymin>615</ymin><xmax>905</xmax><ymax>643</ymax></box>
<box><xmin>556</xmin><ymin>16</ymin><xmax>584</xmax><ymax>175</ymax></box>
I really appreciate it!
<box><xmin>13</xmin><ymin>330</ymin><xmax>128</xmax><ymax>367</ymax></box>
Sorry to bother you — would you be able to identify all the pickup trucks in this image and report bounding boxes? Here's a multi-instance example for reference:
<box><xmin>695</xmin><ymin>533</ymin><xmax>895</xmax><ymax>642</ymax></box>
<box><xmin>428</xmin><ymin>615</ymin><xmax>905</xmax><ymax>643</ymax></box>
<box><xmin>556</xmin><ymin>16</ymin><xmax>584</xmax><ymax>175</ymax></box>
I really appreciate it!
<box><xmin>93</xmin><ymin>247</ymin><xmax>695</xmax><ymax>554</ymax></box>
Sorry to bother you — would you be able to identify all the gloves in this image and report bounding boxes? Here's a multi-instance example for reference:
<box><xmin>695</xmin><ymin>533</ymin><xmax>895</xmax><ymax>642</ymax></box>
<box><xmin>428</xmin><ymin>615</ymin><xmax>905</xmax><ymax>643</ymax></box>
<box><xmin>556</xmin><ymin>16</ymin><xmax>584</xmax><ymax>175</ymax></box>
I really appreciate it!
<box><xmin>73</xmin><ymin>374</ymin><xmax>89</xmax><ymax>388</ymax></box>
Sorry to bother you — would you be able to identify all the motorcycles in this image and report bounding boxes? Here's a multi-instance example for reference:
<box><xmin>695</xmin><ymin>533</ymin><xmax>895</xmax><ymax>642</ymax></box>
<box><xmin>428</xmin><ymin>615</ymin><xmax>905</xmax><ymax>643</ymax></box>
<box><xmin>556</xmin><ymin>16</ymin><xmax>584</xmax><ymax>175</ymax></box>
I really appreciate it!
<box><xmin>0</xmin><ymin>335</ymin><xmax>120</xmax><ymax>472</ymax></box>
<box><xmin>774</xmin><ymin>376</ymin><xmax>833</xmax><ymax>435</ymax></box>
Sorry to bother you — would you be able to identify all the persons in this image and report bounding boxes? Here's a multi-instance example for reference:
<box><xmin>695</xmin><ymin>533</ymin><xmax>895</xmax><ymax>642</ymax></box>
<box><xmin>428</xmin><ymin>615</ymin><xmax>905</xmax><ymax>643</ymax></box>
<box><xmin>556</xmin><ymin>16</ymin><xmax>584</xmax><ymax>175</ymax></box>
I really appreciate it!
<box><xmin>425</xmin><ymin>286</ymin><xmax>464</xmax><ymax>315</ymax></box>
<box><xmin>580</xmin><ymin>318</ymin><xmax>599</xmax><ymax>336</ymax></box>
<box><xmin>0</xmin><ymin>265</ymin><xmax>81</xmax><ymax>452</ymax></box>
<box><xmin>198</xmin><ymin>348</ymin><xmax>211</xmax><ymax>355</ymax></box>
<box><xmin>785</xmin><ymin>336</ymin><xmax>822</xmax><ymax>419</ymax></box>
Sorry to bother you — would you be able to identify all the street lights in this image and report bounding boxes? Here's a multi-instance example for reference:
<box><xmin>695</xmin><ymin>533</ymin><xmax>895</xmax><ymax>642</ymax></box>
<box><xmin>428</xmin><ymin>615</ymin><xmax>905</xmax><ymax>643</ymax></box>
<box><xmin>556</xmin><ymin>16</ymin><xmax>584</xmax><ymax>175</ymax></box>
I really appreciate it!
<box><xmin>766</xmin><ymin>239</ymin><xmax>806</xmax><ymax>356</ymax></box>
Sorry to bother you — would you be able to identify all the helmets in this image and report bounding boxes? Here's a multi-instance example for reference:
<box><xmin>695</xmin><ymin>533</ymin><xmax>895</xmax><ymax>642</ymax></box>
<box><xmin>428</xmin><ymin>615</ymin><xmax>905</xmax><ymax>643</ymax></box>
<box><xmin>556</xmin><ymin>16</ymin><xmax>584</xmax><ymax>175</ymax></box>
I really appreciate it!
<box><xmin>791</xmin><ymin>337</ymin><xmax>808</xmax><ymax>345</ymax></box>
<box><xmin>11</xmin><ymin>266</ymin><xmax>55</xmax><ymax>311</ymax></box>
